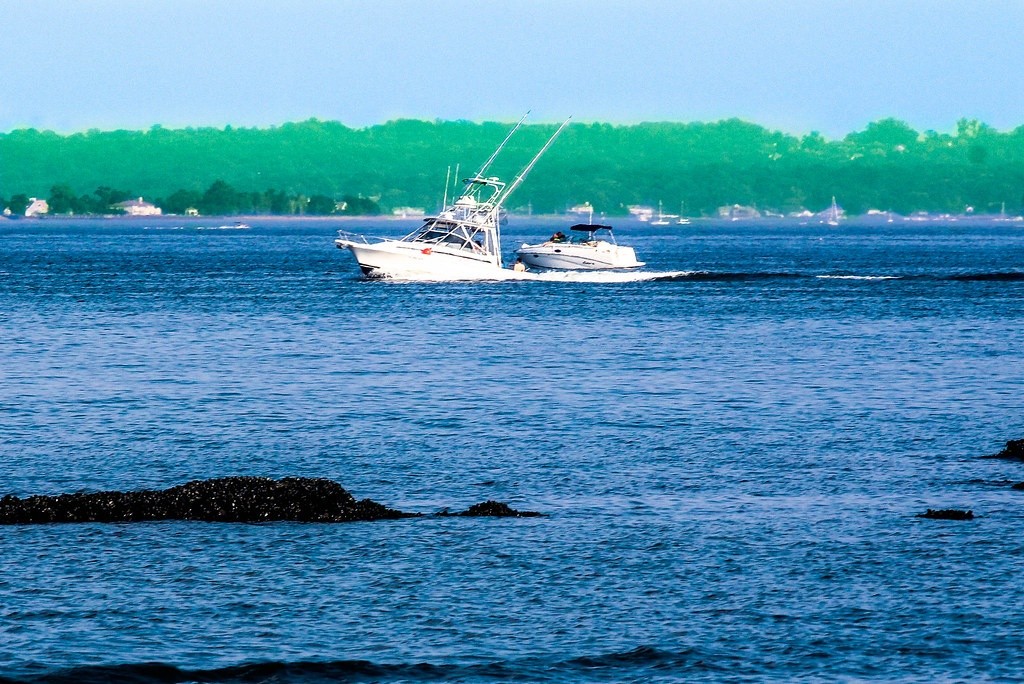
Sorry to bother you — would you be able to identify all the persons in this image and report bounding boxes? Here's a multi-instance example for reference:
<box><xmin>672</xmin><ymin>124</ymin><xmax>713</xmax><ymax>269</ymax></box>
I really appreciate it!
<box><xmin>513</xmin><ymin>258</ymin><xmax>525</xmax><ymax>272</ymax></box>
<box><xmin>550</xmin><ymin>231</ymin><xmax>565</xmax><ymax>242</ymax></box>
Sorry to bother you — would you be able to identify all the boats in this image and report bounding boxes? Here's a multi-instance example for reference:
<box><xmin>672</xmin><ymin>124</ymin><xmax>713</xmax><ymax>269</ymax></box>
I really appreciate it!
<box><xmin>332</xmin><ymin>109</ymin><xmax>576</xmax><ymax>280</ymax></box>
<box><xmin>234</xmin><ymin>221</ymin><xmax>248</xmax><ymax>228</ymax></box>
<box><xmin>387</xmin><ymin>193</ymin><xmax>1024</xmax><ymax>233</ymax></box>
<box><xmin>512</xmin><ymin>224</ymin><xmax>645</xmax><ymax>270</ymax></box>
